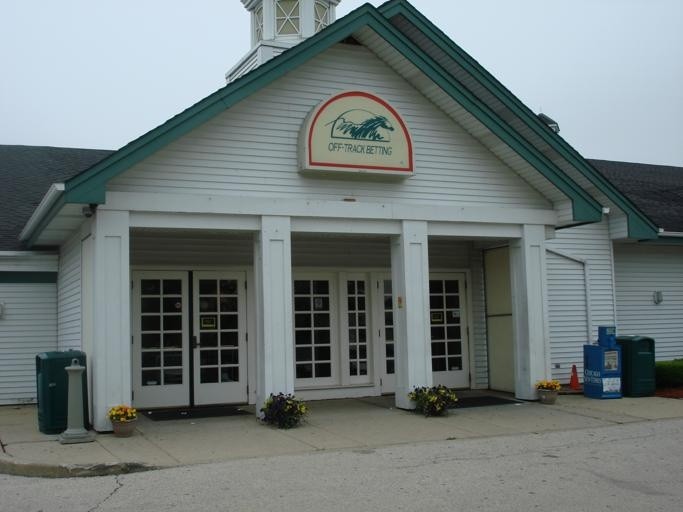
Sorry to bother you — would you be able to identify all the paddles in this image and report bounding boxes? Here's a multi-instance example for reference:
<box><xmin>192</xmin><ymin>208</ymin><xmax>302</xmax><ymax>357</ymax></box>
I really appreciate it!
<box><xmin>448</xmin><ymin>396</ymin><xmax>524</xmax><ymax>408</ymax></box>
<box><xmin>140</xmin><ymin>406</ymin><xmax>255</xmax><ymax>422</ymax></box>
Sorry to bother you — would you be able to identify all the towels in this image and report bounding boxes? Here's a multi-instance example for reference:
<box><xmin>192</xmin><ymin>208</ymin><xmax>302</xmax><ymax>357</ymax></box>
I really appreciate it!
<box><xmin>36</xmin><ymin>350</ymin><xmax>88</xmax><ymax>434</ymax></box>
<box><xmin>582</xmin><ymin>325</ymin><xmax>655</xmax><ymax>399</ymax></box>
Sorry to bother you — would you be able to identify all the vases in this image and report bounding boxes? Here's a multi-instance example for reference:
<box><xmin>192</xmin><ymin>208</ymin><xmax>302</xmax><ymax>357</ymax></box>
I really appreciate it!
<box><xmin>406</xmin><ymin>382</ymin><xmax>459</xmax><ymax>420</ymax></box>
<box><xmin>532</xmin><ymin>377</ymin><xmax>563</xmax><ymax>392</ymax></box>
<box><xmin>256</xmin><ymin>390</ymin><xmax>313</xmax><ymax>432</ymax></box>
<box><xmin>106</xmin><ymin>402</ymin><xmax>138</xmax><ymax>422</ymax></box>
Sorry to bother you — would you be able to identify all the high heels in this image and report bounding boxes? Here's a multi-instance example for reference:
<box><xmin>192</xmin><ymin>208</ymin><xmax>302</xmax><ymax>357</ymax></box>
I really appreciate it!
<box><xmin>566</xmin><ymin>365</ymin><xmax>583</xmax><ymax>390</ymax></box>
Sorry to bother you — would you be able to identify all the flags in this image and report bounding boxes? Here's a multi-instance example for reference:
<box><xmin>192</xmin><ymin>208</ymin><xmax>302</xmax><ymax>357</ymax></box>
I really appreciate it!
<box><xmin>538</xmin><ymin>389</ymin><xmax>557</xmax><ymax>404</ymax></box>
<box><xmin>110</xmin><ymin>418</ymin><xmax>137</xmax><ymax>437</ymax></box>
